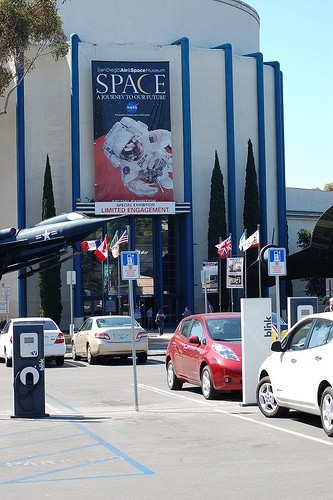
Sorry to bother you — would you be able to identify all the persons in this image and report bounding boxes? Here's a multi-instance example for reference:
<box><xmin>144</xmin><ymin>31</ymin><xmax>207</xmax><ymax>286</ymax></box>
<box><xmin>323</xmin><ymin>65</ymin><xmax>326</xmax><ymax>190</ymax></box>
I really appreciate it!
<box><xmin>105</xmin><ymin>117</ymin><xmax>173</xmax><ymax>196</ymax></box>
<box><xmin>146</xmin><ymin>307</ymin><xmax>153</xmax><ymax>330</ymax></box>
<box><xmin>156</xmin><ymin>309</ymin><xmax>166</xmax><ymax>335</ymax></box>
<box><xmin>324</xmin><ymin>298</ymin><xmax>333</xmax><ymax>312</ymax></box>
<box><xmin>182</xmin><ymin>306</ymin><xmax>191</xmax><ymax>317</ymax></box>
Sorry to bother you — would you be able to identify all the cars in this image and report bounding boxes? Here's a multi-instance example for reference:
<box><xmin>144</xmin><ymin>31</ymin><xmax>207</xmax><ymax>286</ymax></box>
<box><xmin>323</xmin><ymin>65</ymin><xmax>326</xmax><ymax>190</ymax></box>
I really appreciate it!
<box><xmin>255</xmin><ymin>312</ymin><xmax>333</xmax><ymax>438</ymax></box>
<box><xmin>0</xmin><ymin>316</ymin><xmax>66</xmax><ymax>367</ymax></box>
<box><xmin>164</xmin><ymin>311</ymin><xmax>287</xmax><ymax>400</ymax></box>
<box><xmin>71</xmin><ymin>315</ymin><xmax>149</xmax><ymax>365</ymax></box>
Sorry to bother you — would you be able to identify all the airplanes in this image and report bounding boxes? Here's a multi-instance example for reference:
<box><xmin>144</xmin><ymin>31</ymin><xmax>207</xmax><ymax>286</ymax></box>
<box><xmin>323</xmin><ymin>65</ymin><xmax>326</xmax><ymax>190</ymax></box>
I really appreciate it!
<box><xmin>0</xmin><ymin>210</ymin><xmax>127</xmax><ymax>281</ymax></box>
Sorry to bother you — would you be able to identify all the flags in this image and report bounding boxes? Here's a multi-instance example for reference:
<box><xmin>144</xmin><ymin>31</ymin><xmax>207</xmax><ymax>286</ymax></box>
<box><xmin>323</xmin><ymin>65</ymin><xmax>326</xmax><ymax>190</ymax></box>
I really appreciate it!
<box><xmin>215</xmin><ymin>236</ymin><xmax>232</xmax><ymax>259</ymax></box>
<box><xmin>239</xmin><ymin>230</ymin><xmax>259</xmax><ymax>251</ymax></box>
<box><xmin>80</xmin><ymin>230</ymin><xmax>129</xmax><ymax>261</ymax></box>
